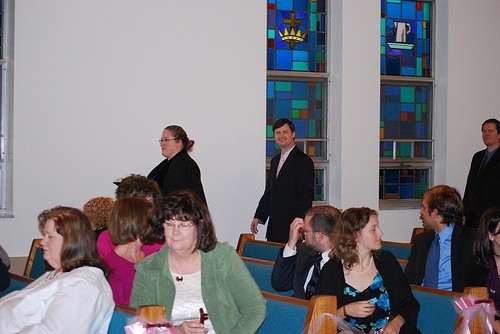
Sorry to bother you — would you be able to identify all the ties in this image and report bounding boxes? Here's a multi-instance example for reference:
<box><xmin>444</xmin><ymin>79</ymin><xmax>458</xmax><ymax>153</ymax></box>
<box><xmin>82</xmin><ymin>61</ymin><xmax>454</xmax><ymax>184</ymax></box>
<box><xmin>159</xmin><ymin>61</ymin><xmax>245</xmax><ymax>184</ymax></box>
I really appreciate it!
<box><xmin>423</xmin><ymin>233</ymin><xmax>440</xmax><ymax>288</ymax></box>
<box><xmin>306</xmin><ymin>254</ymin><xmax>322</xmax><ymax>300</ymax></box>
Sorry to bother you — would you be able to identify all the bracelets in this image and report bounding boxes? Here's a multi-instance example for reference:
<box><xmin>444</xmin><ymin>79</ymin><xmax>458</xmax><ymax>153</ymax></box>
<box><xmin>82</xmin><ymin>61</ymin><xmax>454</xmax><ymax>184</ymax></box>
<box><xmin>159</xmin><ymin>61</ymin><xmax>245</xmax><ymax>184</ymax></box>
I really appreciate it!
<box><xmin>343</xmin><ymin>305</ymin><xmax>349</xmax><ymax>318</ymax></box>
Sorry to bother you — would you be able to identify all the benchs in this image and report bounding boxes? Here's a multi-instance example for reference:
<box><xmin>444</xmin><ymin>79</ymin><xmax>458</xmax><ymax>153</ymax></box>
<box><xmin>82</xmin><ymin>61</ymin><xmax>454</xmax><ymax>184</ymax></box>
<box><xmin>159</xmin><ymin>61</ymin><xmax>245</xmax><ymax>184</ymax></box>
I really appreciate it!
<box><xmin>0</xmin><ymin>234</ymin><xmax>489</xmax><ymax>334</ymax></box>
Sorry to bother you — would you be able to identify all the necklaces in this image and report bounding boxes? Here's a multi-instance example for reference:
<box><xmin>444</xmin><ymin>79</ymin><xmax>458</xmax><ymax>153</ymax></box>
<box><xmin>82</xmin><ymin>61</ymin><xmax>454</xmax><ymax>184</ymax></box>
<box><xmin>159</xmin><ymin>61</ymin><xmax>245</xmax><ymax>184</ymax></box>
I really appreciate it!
<box><xmin>494</xmin><ymin>253</ymin><xmax>500</xmax><ymax>258</ymax></box>
<box><xmin>171</xmin><ymin>256</ymin><xmax>198</xmax><ymax>275</ymax></box>
<box><xmin>352</xmin><ymin>256</ymin><xmax>373</xmax><ymax>273</ymax></box>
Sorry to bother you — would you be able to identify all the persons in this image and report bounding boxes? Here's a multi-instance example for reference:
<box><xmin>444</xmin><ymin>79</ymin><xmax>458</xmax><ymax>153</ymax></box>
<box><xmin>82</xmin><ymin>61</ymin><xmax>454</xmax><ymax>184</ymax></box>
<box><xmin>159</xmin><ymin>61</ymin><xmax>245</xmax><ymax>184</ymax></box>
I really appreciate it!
<box><xmin>146</xmin><ymin>125</ymin><xmax>208</xmax><ymax>213</ymax></box>
<box><xmin>461</xmin><ymin>118</ymin><xmax>500</xmax><ymax>243</ymax></box>
<box><xmin>113</xmin><ymin>173</ymin><xmax>166</xmax><ymax>246</ymax></box>
<box><xmin>405</xmin><ymin>185</ymin><xmax>483</xmax><ymax>295</ymax></box>
<box><xmin>313</xmin><ymin>207</ymin><xmax>421</xmax><ymax>334</ymax></box>
<box><xmin>0</xmin><ymin>205</ymin><xmax>118</xmax><ymax>334</ymax></box>
<box><xmin>127</xmin><ymin>192</ymin><xmax>266</xmax><ymax>334</ymax></box>
<box><xmin>82</xmin><ymin>197</ymin><xmax>115</xmax><ymax>281</ymax></box>
<box><xmin>0</xmin><ymin>246</ymin><xmax>11</xmax><ymax>293</ymax></box>
<box><xmin>271</xmin><ymin>205</ymin><xmax>343</xmax><ymax>301</ymax></box>
<box><xmin>96</xmin><ymin>196</ymin><xmax>164</xmax><ymax>308</ymax></box>
<box><xmin>472</xmin><ymin>207</ymin><xmax>500</xmax><ymax>334</ymax></box>
<box><xmin>250</xmin><ymin>118</ymin><xmax>315</xmax><ymax>245</ymax></box>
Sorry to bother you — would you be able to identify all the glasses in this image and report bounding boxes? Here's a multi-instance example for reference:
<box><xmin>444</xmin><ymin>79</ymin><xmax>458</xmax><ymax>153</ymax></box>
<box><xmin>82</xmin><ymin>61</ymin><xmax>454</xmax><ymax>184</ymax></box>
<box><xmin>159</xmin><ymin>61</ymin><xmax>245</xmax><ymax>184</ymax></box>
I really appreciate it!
<box><xmin>299</xmin><ymin>228</ymin><xmax>326</xmax><ymax>236</ymax></box>
<box><xmin>158</xmin><ymin>139</ymin><xmax>178</xmax><ymax>142</ymax></box>
<box><xmin>162</xmin><ymin>222</ymin><xmax>196</xmax><ymax>230</ymax></box>
